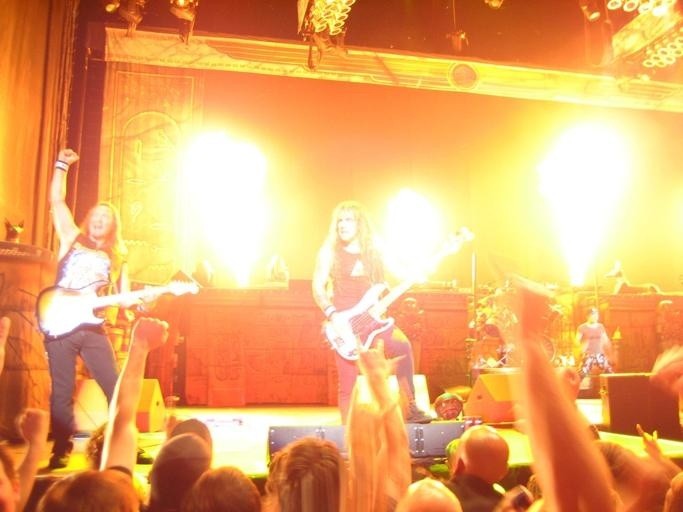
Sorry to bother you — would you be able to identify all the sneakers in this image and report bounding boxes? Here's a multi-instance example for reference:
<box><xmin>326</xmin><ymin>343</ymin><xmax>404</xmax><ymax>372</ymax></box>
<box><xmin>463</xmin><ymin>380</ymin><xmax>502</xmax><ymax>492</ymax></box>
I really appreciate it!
<box><xmin>50</xmin><ymin>448</ymin><xmax>71</xmax><ymax>467</ymax></box>
<box><xmin>404</xmin><ymin>408</ymin><xmax>433</xmax><ymax>424</ymax></box>
<box><xmin>137</xmin><ymin>447</ymin><xmax>154</xmax><ymax>464</ymax></box>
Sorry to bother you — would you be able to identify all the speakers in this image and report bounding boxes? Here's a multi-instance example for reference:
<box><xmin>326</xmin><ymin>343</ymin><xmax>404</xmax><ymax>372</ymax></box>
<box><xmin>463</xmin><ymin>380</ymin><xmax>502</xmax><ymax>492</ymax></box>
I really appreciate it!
<box><xmin>462</xmin><ymin>372</ymin><xmax>525</xmax><ymax>423</ymax></box>
<box><xmin>72</xmin><ymin>378</ymin><xmax>166</xmax><ymax>434</ymax></box>
<box><xmin>404</xmin><ymin>418</ymin><xmax>482</xmax><ymax>459</ymax></box>
<box><xmin>268</xmin><ymin>425</ymin><xmax>350</xmax><ymax>461</ymax></box>
<box><xmin>599</xmin><ymin>373</ymin><xmax>680</xmax><ymax>440</ymax></box>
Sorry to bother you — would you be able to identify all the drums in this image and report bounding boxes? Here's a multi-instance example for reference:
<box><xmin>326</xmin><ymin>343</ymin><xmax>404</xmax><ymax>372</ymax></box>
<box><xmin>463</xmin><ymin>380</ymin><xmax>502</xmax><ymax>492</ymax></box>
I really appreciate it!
<box><xmin>470</xmin><ymin>322</ymin><xmax>553</xmax><ymax>388</ymax></box>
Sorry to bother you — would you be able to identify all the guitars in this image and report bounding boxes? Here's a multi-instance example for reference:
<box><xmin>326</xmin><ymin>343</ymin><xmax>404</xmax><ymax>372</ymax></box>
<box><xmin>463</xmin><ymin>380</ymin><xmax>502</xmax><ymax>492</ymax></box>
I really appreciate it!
<box><xmin>36</xmin><ymin>279</ymin><xmax>199</xmax><ymax>340</ymax></box>
<box><xmin>321</xmin><ymin>241</ymin><xmax>459</xmax><ymax>362</ymax></box>
